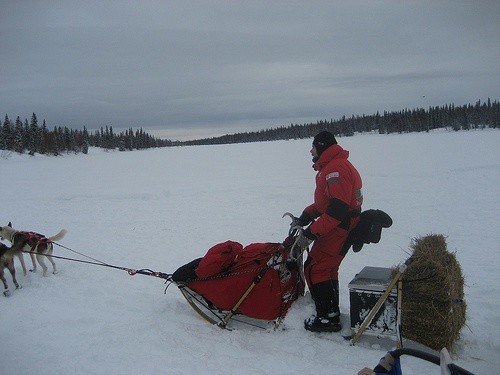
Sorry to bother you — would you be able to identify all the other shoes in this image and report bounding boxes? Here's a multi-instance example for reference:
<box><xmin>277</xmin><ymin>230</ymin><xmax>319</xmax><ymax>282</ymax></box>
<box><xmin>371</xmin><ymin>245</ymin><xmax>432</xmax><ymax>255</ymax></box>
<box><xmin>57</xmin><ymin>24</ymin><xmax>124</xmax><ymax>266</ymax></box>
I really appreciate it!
<box><xmin>304</xmin><ymin>314</ymin><xmax>343</xmax><ymax>332</ymax></box>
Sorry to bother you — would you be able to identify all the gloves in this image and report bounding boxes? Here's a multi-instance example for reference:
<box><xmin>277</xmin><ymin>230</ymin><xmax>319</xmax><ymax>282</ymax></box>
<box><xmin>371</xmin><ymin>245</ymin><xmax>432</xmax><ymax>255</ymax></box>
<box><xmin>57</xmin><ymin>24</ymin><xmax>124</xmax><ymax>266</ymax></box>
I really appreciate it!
<box><xmin>290</xmin><ymin>217</ymin><xmax>304</xmax><ymax>230</ymax></box>
<box><xmin>297</xmin><ymin>235</ymin><xmax>312</xmax><ymax>250</ymax></box>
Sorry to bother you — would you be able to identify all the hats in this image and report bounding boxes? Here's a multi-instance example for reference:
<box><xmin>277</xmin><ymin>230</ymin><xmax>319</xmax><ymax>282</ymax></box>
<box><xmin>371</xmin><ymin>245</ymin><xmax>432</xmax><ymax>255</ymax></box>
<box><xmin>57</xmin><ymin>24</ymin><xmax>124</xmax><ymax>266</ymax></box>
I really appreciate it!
<box><xmin>314</xmin><ymin>130</ymin><xmax>337</xmax><ymax>158</ymax></box>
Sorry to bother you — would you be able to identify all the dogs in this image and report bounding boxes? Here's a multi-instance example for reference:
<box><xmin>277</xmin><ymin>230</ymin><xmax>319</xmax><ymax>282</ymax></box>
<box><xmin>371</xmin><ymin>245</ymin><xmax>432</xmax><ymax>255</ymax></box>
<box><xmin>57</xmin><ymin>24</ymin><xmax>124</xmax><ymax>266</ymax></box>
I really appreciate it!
<box><xmin>0</xmin><ymin>241</ymin><xmax>24</xmax><ymax>296</ymax></box>
<box><xmin>0</xmin><ymin>222</ymin><xmax>67</xmax><ymax>277</ymax></box>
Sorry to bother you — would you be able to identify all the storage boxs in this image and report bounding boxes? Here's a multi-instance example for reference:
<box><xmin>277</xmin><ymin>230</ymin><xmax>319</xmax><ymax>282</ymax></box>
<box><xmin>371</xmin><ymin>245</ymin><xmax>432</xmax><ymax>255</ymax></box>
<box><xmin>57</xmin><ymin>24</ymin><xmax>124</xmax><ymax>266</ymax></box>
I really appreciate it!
<box><xmin>348</xmin><ymin>266</ymin><xmax>399</xmax><ymax>337</ymax></box>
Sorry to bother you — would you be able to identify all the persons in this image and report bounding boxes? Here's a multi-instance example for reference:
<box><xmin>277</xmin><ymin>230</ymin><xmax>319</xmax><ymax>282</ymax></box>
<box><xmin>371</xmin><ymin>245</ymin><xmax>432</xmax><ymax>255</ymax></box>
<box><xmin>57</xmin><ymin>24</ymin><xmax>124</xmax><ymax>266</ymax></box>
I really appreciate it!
<box><xmin>284</xmin><ymin>131</ymin><xmax>363</xmax><ymax>333</ymax></box>
